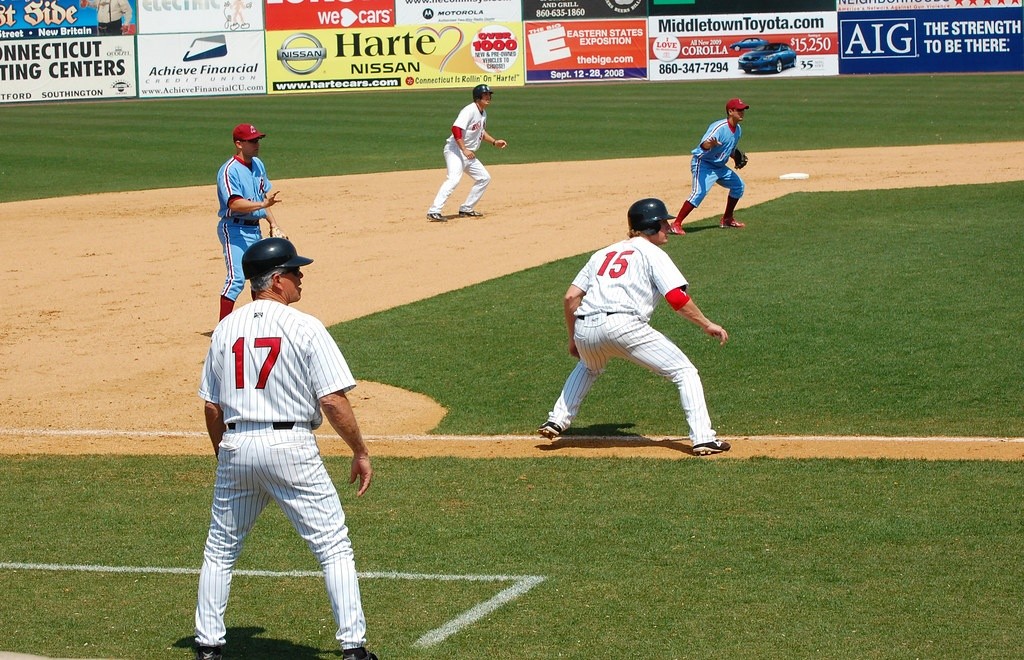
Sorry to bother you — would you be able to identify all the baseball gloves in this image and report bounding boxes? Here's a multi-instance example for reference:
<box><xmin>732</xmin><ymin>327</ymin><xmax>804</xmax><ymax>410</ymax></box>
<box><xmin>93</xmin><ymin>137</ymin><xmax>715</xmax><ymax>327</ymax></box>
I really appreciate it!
<box><xmin>270</xmin><ymin>227</ymin><xmax>289</xmax><ymax>240</ymax></box>
<box><xmin>734</xmin><ymin>148</ymin><xmax>748</xmax><ymax>169</ymax></box>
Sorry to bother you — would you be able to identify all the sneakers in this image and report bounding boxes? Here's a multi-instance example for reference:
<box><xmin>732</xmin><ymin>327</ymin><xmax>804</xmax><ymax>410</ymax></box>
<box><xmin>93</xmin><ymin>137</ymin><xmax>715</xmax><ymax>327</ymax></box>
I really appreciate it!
<box><xmin>537</xmin><ymin>421</ymin><xmax>561</xmax><ymax>441</ymax></box>
<box><xmin>667</xmin><ymin>222</ymin><xmax>685</xmax><ymax>235</ymax></box>
<box><xmin>458</xmin><ymin>211</ymin><xmax>483</xmax><ymax>218</ymax></box>
<box><xmin>692</xmin><ymin>438</ymin><xmax>730</xmax><ymax>456</ymax></box>
<box><xmin>427</xmin><ymin>213</ymin><xmax>446</xmax><ymax>223</ymax></box>
<box><xmin>720</xmin><ymin>219</ymin><xmax>746</xmax><ymax>230</ymax></box>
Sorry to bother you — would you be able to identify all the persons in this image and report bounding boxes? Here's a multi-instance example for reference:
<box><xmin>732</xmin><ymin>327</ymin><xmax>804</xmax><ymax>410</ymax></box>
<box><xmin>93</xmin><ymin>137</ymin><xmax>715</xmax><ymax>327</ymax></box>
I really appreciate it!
<box><xmin>216</xmin><ymin>122</ymin><xmax>286</xmax><ymax>323</ymax></box>
<box><xmin>669</xmin><ymin>99</ymin><xmax>749</xmax><ymax>236</ymax></box>
<box><xmin>193</xmin><ymin>237</ymin><xmax>380</xmax><ymax>660</ymax></box>
<box><xmin>428</xmin><ymin>85</ymin><xmax>508</xmax><ymax>222</ymax></box>
<box><xmin>81</xmin><ymin>0</ymin><xmax>133</xmax><ymax>37</ymax></box>
<box><xmin>537</xmin><ymin>202</ymin><xmax>733</xmax><ymax>456</ymax></box>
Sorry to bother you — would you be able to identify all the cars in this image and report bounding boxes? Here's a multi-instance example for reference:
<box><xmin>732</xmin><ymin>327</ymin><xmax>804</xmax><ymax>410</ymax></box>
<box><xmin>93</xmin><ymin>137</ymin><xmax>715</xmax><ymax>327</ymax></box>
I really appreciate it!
<box><xmin>737</xmin><ymin>42</ymin><xmax>798</xmax><ymax>73</ymax></box>
<box><xmin>729</xmin><ymin>38</ymin><xmax>768</xmax><ymax>52</ymax></box>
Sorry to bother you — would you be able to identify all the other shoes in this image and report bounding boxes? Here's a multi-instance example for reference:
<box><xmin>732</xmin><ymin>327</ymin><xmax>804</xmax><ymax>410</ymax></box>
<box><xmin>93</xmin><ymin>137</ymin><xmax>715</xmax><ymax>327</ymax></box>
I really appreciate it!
<box><xmin>203</xmin><ymin>645</ymin><xmax>224</xmax><ymax>660</ymax></box>
<box><xmin>343</xmin><ymin>647</ymin><xmax>378</xmax><ymax>660</ymax></box>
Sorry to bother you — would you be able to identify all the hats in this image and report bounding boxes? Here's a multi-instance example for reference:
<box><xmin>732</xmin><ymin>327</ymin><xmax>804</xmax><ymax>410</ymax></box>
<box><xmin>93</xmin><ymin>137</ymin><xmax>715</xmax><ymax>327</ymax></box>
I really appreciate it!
<box><xmin>233</xmin><ymin>124</ymin><xmax>266</xmax><ymax>142</ymax></box>
<box><xmin>725</xmin><ymin>99</ymin><xmax>750</xmax><ymax>113</ymax></box>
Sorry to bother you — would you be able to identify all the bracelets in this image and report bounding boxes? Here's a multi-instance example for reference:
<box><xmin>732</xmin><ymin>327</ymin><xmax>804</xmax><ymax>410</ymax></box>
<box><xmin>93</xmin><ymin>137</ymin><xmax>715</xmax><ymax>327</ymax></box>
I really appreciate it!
<box><xmin>492</xmin><ymin>139</ymin><xmax>496</xmax><ymax>146</ymax></box>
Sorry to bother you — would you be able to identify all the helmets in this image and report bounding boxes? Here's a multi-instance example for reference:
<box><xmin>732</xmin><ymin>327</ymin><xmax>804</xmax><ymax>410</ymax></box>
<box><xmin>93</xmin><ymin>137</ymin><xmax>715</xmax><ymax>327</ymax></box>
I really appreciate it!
<box><xmin>242</xmin><ymin>237</ymin><xmax>314</xmax><ymax>280</ymax></box>
<box><xmin>473</xmin><ymin>84</ymin><xmax>493</xmax><ymax>98</ymax></box>
<box><xmin>627</xmin><ymin>198</ymin><xmax>676</xmax><ymax>236</ymax></box>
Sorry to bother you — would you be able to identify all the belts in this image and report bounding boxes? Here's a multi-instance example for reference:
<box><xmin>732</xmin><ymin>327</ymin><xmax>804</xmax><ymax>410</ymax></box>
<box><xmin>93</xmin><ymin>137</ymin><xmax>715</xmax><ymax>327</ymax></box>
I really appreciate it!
<box><xmin>228</xmin><ymin>422</ymin><xmax>298</xmax><ymax>431</ymax></box>
<box><xmin>232</xmin><ymin>218</ymin><xmax>260</xmax><ymax>226</ymax></box>
<box><xmin>577</xmin><ymin>312</ymin><xmax>612</xmax><ymax>320</ymax></box>
